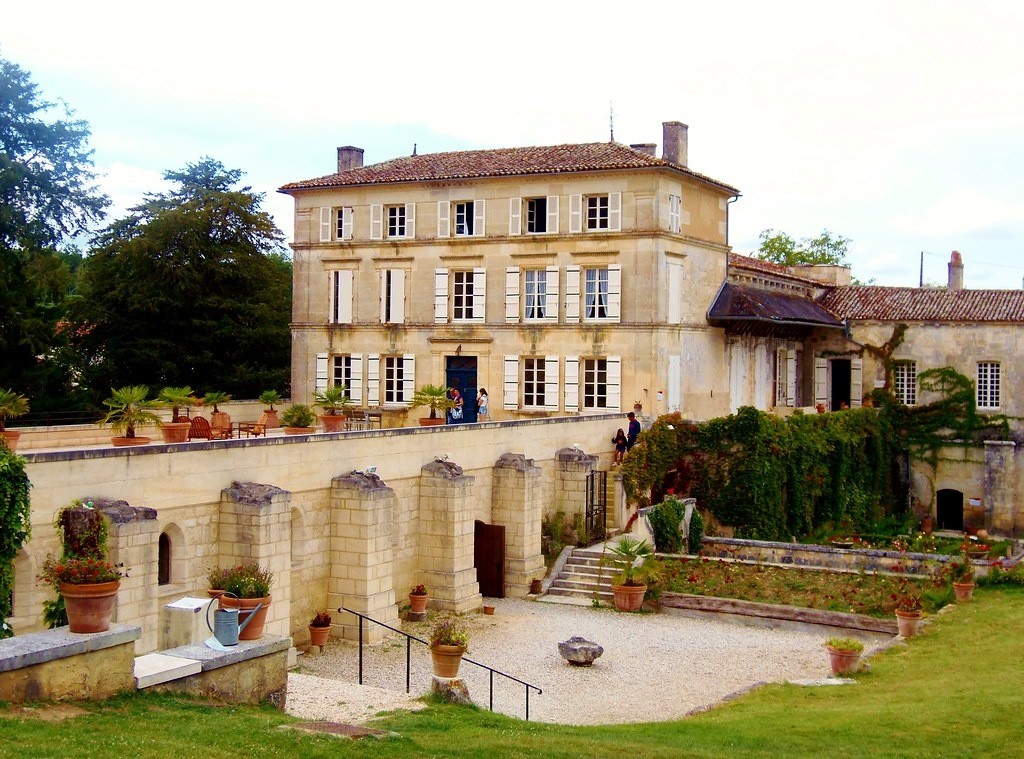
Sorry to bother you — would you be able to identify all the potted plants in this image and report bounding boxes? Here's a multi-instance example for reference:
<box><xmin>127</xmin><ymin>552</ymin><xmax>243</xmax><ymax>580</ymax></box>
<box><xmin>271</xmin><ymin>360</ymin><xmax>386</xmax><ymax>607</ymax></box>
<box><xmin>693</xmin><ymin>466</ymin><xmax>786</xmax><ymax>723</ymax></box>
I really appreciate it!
<box><xmin>0</xmin><ymin>383</ymin><xmax>457</xmax><ymax>453</ymax></box>
<box><xmin>825</xmin><ymin>636</ymin><xmax>864</xmax><ymax>676</ymax></box>
<box><xmin>602</xmin><ymin>535</ymin><xmax>665</xmax><ymax>612</ymax></box>
<box><xmin>309</xmin><ymin>610</ymin><xmax>331</xmax><ymax>646</ymax></box>
<box><xmin>953</xmin><ymin>580</ymin><xmax>974</xmax><ymax>601</ymax></box>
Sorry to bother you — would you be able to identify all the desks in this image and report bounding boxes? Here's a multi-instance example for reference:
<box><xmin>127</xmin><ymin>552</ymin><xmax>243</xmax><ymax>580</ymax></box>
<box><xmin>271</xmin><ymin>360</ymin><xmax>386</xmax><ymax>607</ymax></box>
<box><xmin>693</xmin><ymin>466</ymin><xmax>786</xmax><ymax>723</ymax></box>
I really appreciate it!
<box><xmin>230</xmin><ymin>420</ymin><xmax>268</xmax><ymax>438</ymax></box>
<box><xmin>336</xmin><ymin>410</ymin><xmax>382</xmax><ymax>429</ymax></box>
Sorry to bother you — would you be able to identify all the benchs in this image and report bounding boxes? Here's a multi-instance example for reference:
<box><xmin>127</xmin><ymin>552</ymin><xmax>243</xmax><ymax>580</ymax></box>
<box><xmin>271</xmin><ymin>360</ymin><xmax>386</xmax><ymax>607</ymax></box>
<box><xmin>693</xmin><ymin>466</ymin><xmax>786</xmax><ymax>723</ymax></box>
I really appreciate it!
<box><xmin>177</xmin><ymin>415</ymin><xmax>223</xmax><ymax>440</ymax></box>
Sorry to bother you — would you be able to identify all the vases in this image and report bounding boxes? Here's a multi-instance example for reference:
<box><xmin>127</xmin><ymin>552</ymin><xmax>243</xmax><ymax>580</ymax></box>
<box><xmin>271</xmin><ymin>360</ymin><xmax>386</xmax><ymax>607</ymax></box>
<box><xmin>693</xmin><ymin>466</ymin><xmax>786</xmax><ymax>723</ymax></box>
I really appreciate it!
<box><xmin>895</xmin><ymin>608</ymin><xmax>923</xmax><ymax>636</ymax></box>
<box><xmin>206</xmin><ymin>590</ymin><xmax>272</xmax><ymax>640</ymax></box>
<box><xmin>60</xmin><ymin>582</ymin><xmax>121</xmax><ymax>633</ymax></box>
<box><xmin>409</xmin><ymin>593</ymin><xmax>429</xmax><ymax>612</ymax></box>
<box><xmin>432</xmin><ymin>646</ymin><xmax>464</xmax><ymax>677</ymax></box>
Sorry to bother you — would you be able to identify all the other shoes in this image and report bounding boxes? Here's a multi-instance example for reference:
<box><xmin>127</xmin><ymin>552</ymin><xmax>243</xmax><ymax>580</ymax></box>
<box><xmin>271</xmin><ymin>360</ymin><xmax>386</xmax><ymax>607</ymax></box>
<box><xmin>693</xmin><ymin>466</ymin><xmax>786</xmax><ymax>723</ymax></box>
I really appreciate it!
<box><xmin>612</xmin><ymin>462</ymin><xmax>617</xmax><ymax>467</ymax></box>
<box><xmin>619</xmin><ymin>462</ymin><xmax>622</xmax><ymax>465</ymax></box>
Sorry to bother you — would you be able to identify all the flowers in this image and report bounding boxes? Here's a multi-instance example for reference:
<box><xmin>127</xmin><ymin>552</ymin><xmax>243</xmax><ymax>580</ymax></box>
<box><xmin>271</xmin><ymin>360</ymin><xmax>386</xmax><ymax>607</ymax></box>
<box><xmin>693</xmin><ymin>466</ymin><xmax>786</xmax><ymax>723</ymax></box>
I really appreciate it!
<box><xmin>898</xmin><ymin>592</ymin><xmax>922</xmax><ymax>613</ymax></box>
<box><xmin>952</xmin><ymin>560</ymin><xmax>975</xmax><ymax>582</ymax></box>
<box><xmin>427</xmin><ymin>618</ymin><xmax>469</xmax><ymax>646</ymax></box>
<box><xmin>39</xmin><ymin>553</ymin><xmax>131</xmax><ymax>582</ymax></box>
<box><xmin>410</xmin><ymin>583</ymin><xmax>428</xmax><ymax>595</ymax></box>
<box><xmin>208</xmin><ymin>565</ymin><xmax>273</xmax><ymax>597</ymax></box>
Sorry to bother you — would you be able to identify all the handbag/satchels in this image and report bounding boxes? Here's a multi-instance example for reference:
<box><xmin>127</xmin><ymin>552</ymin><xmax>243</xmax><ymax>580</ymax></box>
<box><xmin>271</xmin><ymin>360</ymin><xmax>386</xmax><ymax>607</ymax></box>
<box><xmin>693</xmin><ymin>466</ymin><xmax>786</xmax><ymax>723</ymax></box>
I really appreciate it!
<box><xmin>452</xmin><ymin>408</ymin><xmax>462</xmax><ymax>419</ymax></box>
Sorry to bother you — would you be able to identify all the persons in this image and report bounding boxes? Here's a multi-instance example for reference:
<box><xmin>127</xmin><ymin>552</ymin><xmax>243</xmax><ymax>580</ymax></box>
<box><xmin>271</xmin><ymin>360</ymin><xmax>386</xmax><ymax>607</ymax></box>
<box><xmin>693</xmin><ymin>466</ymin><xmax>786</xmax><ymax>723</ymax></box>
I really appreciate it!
<box><xmin>478</xmin><ymin>387</ymin><xmax>488</xmax><ymax>423</ymax></box>
<box><xmin>611</xmin><ymin>428</ymin><xmax>628</xmax><ymax>466</ymax></box>
<box><xmin>447</xmin><ymin>387</ymin><xmax>463</xmax><ymax>424</ymax></box>
<box><xmin>627</xmin><ymin>412</ymin><xmax>641</xmax><ymax>452</ymax></box>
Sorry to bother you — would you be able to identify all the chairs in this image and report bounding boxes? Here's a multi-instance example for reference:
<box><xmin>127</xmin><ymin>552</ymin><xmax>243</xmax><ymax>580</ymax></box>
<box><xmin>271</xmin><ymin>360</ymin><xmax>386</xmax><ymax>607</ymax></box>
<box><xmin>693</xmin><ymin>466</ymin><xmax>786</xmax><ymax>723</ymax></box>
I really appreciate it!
<box><xmin>341</xmin><ymin>409</ymin><xmax>373</xmax><ymax>431</ymax></box>
